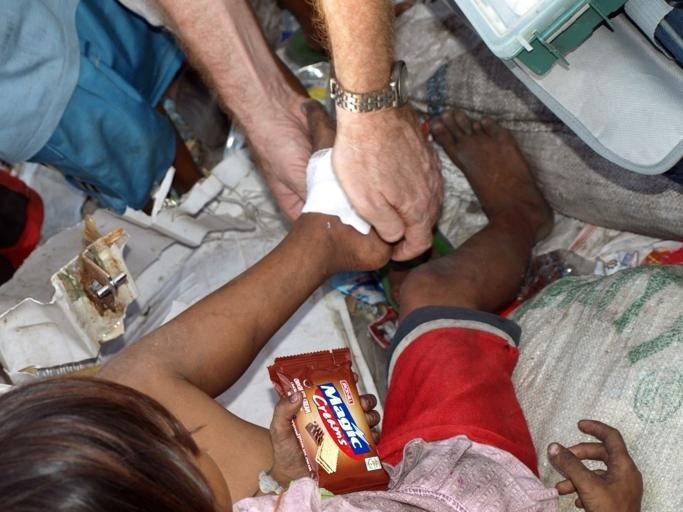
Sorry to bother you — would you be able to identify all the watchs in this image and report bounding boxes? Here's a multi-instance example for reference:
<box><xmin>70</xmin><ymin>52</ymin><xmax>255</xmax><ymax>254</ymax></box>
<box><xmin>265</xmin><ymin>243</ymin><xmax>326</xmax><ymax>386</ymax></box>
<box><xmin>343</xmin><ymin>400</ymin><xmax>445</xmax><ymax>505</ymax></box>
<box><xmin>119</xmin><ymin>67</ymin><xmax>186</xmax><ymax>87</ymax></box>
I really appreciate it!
<box><xmin>327</xmin><ymin>60</ymin><xmax>408</xmax><ymax>113</ymax></box>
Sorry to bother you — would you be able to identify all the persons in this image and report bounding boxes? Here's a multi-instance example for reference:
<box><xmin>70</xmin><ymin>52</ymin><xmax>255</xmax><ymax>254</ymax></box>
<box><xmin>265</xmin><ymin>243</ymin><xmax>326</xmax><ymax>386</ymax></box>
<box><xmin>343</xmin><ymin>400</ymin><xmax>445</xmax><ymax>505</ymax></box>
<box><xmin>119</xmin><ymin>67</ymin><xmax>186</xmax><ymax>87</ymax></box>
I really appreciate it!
<box><xmin>157</xmin><ymin>0</ymin><xmax>442</xmax><ymax>262</ymax></box>
<box><xmin>0</xmin><ymin>0</ymin><xmax>205</xmax><ymax>217</ymax></box>
<box><xmin>0</xmin><ymin>106</ymin><xmax>643</xmax><ymax>512</ymax></box>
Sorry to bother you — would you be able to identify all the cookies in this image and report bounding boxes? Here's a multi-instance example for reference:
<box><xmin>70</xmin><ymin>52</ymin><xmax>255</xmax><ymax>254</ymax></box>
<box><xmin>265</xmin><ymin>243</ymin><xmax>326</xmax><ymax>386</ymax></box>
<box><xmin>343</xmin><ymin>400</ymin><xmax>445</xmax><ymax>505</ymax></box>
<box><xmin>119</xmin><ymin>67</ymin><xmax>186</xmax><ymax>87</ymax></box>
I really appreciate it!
<box><xmin>306</xmin><ymin>420</ymin><xmax>338</xmax><ymax>474</ymax></box>
